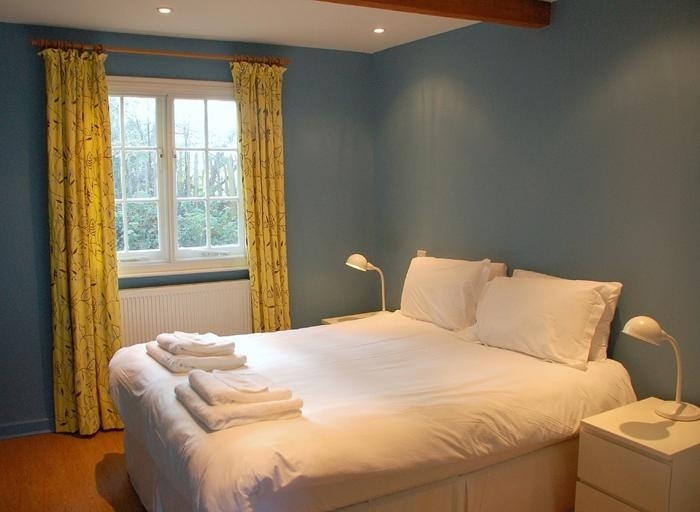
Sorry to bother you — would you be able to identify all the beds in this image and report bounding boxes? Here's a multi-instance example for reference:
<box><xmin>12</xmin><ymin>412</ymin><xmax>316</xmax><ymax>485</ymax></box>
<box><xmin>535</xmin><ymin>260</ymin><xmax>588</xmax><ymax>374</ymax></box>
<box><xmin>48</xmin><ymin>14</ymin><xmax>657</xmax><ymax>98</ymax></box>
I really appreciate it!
<box><xmin>106</xmin><ymin>310</ymin><xmax>637</xmax><ymax>512</ymax></box>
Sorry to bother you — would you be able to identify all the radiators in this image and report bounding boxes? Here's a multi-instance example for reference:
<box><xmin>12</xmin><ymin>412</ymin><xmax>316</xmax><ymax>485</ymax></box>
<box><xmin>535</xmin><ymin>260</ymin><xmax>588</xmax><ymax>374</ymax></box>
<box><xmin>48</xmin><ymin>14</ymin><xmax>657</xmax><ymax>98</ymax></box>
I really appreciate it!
<box><xmin>118</xmin><ymin>280</ymin><xmax>254</xmax><ymax>348</ymax></box>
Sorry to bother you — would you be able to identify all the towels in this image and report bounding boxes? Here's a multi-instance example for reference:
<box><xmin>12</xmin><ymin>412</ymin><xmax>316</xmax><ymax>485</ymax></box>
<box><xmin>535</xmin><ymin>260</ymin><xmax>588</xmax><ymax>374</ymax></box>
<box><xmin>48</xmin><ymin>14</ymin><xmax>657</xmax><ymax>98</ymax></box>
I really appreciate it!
<box><xmin>146</xmin><ymin>331</ymin><xmax>305</xmax><ymax>432</ymax></box>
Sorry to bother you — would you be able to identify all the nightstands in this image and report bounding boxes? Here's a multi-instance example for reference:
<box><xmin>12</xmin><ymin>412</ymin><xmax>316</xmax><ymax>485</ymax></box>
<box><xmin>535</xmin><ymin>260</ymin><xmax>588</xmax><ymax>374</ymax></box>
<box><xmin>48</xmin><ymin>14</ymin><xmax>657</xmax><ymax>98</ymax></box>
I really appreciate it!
<box><xmin>321</xmin><ymin>310</ymin><xmax>393</xmax><ymax>325</ymax></box>
<box><xmin>574</xmin><ymin>396</ymin><xmax>700</xmax><ymax>512</ymax></box>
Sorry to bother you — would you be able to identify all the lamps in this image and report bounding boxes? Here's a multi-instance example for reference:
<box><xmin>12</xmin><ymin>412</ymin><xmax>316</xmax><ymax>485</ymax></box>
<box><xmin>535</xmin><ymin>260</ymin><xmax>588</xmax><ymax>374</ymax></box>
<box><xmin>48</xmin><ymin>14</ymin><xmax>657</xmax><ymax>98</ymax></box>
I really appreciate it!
<box><xmin>622</xmin><ymin>315</ymin><xmax>700</xmax><ymax>421</ymax></box>
<box><xmin>346</xmin><ymin>253</ymin><xmax>391</xmax><ymax>315</ymax></box>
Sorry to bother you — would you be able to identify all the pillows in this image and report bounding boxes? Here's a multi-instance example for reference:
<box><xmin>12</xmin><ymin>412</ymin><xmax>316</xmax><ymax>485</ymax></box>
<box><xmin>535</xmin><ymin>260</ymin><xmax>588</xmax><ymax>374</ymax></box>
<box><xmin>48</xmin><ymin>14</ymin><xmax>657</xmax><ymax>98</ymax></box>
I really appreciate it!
<box><xmin>400</xmin><ymin>256</ymin><xmax>623</xmax><ymax>373</ymax></box>
